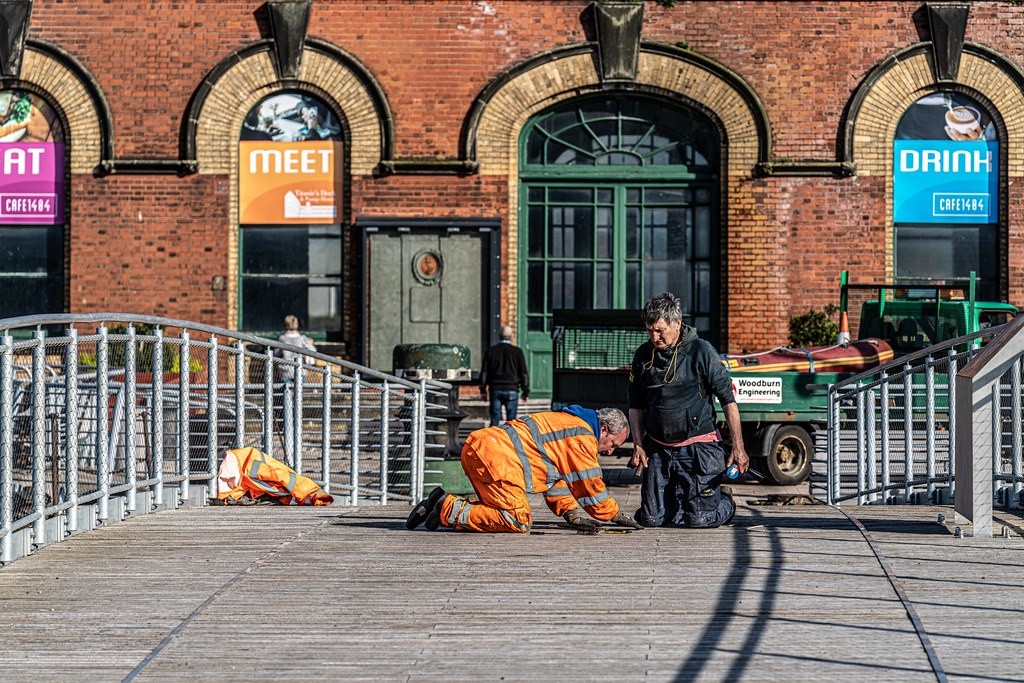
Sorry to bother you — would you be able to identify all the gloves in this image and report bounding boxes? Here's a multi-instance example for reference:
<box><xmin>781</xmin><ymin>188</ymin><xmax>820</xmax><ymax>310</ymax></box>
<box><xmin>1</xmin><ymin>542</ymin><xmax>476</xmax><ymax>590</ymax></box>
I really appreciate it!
<box><xmin>573</xmin><ymin>516</ymin><xmax>604</xmax><ymax>535</ymax></box>
<box><xmin>616</xmin><ymin>512</ymin><xmax>644</xmax><ymax>530</ymax></box>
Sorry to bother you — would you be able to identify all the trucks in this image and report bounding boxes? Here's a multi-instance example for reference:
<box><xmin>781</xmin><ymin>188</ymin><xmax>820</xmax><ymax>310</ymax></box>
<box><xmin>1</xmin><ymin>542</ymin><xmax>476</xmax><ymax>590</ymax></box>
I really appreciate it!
<box><xmin>550</xmin><ymin>270</ymin><xmax>1023</xmax><ymax>487</ymax></box>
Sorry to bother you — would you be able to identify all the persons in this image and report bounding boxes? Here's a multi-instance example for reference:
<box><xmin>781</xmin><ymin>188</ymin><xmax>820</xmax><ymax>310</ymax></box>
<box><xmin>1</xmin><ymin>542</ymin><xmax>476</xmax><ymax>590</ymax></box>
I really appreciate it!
<box><xmin>406</xmin><ymin>404</ymin><xmax>645</xmax><ymax>532</ymax></box>
<box><xmin>478</xmin><ymin>326</ymin><xmax>531</xmax><ymax>427</ymax></box>
<box><xmin>274</xmin><ymin>314</ymin><xmax>317</xmax><ymax>384</ymax></box>
<box><xmin>944</xmin><ymin>122</ymin><xmax>998</xmax><ymax>141</ymax></box>
<box><xmin>629</xmin><ymin>295</ymin><xmax>749</xmax><ymax>527</ymax></box>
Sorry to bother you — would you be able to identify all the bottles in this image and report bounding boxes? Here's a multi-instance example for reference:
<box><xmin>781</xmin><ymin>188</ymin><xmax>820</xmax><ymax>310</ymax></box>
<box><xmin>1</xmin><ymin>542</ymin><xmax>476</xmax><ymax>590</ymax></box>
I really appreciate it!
<box><xmin>726</xmin><ymin>464</ymin><xmax>740</xmax><ymax>479</ymax></box>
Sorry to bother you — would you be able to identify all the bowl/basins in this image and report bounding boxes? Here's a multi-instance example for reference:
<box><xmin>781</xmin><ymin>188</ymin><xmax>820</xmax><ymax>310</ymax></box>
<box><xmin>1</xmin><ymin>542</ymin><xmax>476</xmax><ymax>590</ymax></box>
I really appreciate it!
<box><xmin>944</xmin><ymin>106</ymin><xmax>981</xmax><ymax>134</ymax></box>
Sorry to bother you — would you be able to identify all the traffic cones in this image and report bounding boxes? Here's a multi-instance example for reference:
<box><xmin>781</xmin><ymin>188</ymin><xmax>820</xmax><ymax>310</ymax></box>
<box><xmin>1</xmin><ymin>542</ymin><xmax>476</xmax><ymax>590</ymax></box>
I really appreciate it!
<box><xmin>837</xmin><ymin>310</ymin><xmax>850</xmax><ymax>344</ymax></box>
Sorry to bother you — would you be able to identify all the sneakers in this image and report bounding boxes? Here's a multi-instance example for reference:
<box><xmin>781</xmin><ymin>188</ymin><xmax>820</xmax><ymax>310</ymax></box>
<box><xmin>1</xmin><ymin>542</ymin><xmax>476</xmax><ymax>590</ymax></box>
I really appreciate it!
<box><xmin>405</xmin><ymin>486</ymin><xmax>445</xmax><ymax>530</ymax></box>
<box><xmin>720</xmin><ymin>486</ymin><xmax>736</xmax><ymax>525</ymax></box>
<box><xmin>425</xmin><ymin>492</ymin><xmax>449</xmax><ymax>531</ymax></box>
